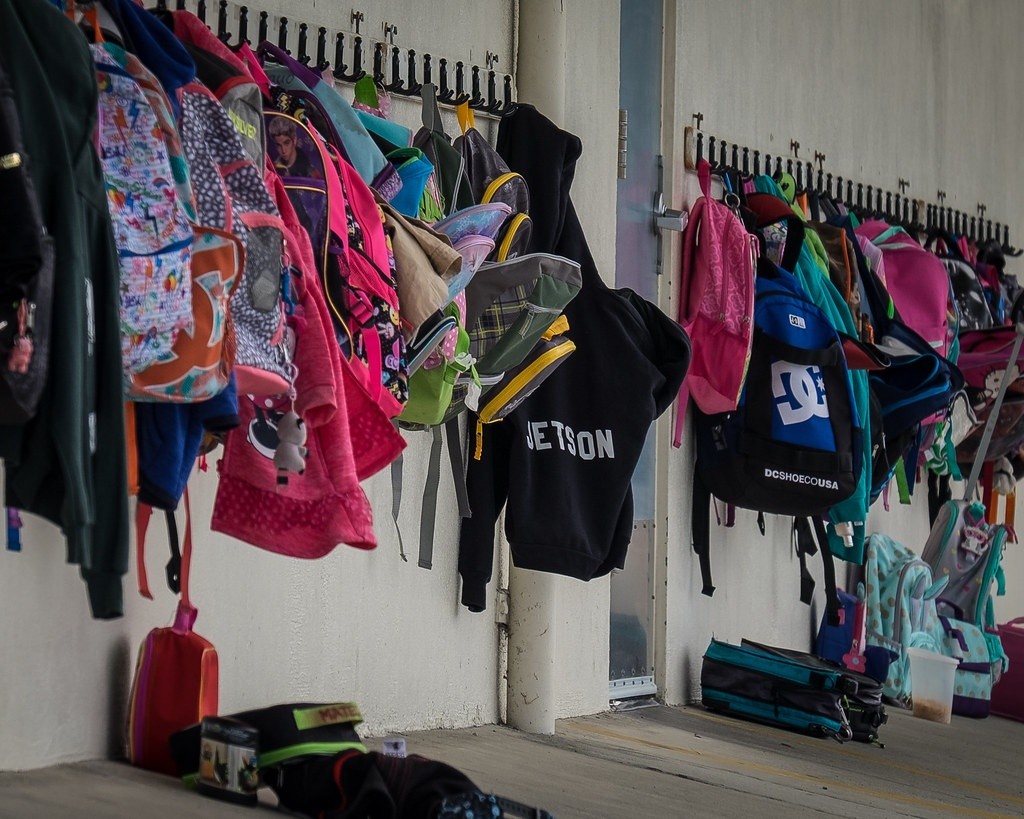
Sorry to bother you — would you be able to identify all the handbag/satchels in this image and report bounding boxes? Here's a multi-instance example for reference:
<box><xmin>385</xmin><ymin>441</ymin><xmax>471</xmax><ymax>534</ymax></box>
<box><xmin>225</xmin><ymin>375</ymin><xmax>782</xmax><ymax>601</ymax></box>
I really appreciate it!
<box><xmin>701</xmin><ymin>637</ymin><xmax>888</xmax><ymax>742</ymax></box>
<box><xmin>935</xmin><ymin>597</ymin><xmax>991</xmax><ymax>719</ymax></box>
<box><xmin>986</xmin><ymin>617</ymin><xmax>1024</xmax><ymax>722</ymax></box>
<box><xmin>169</xmin><ymin>703</ymin><xmax>368</xmax><ymax>809</ymax></box>
<box><xmin>124</xmin><ymin>600</ymin><xmax>218</xmax><ymax>779</ymax></box>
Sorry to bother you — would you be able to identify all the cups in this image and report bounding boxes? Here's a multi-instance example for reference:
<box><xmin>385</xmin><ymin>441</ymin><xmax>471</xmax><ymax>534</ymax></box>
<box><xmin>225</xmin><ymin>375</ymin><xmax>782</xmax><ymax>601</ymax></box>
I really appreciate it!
<box><xmin>907</xmin><ymin>647</ymin><xmax>958</xmax><ymax>723</ymax></box>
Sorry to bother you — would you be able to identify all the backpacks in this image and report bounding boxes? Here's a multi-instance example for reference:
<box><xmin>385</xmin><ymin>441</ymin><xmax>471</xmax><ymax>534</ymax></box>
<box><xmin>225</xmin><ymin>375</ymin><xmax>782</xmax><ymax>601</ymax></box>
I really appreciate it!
<box><xmin>920</xmin><ymin>499</ymin><xmax>1018</xmax><ymax>685</ymax></box>
<box><xmin>681</xmin><ymin>157</ymin><xmax>1024</xmax><ymax>515</ymax></box>
<box><xmin>0</xmin><ymin>0</ymin><xmax>582</xmax><ymax>431</ymax></box>
<box><xmin>816</xmin><ymin>588</ymin><xmax>900</xmax><ymax>683</ymax></box>
<box><xmin>857</xmin><ymin>532</ymin><xmax>951</xmax><ymax>710</ymax></box>
